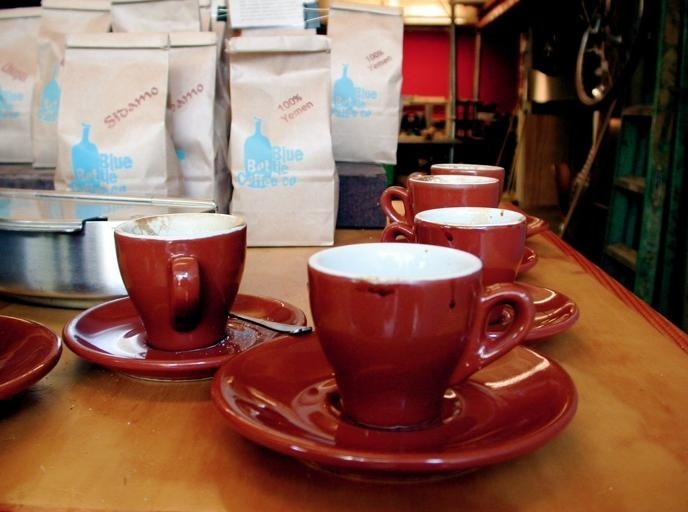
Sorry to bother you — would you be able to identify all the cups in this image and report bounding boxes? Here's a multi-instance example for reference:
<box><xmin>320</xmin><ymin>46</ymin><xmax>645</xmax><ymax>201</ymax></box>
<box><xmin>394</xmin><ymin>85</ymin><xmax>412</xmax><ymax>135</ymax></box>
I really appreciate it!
<box><xmin>114</xmin><ymin>211</ymin><xmax>248</xmax><ymax>349</ymax></box>
<box><xmin>380</xmin><ymin>208</ymin><xmax>529</xmax><ymax>287</ymax></box>
<box><xmin>428</xmin><ymin>162</ymin><xmax>506</xmax><ymax>179</ymax></box>
<box><xmin>380</xmin><ymin>171</ymin><xmax>503</xmax><ymax>221</ymax></box>
<box><xmin>306</xmin><ymin>241</ymin><xmax>534</xmax><ymax>431</ymax></box>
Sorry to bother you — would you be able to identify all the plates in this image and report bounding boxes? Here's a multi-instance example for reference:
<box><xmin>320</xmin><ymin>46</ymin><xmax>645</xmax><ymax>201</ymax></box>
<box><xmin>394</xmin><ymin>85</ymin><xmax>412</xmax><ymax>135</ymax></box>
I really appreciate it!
<box><xmin>519</xmin><ymin>215</ymin><xmax>551</xmax><ymax>276</ymax></box>
<box><xmin>0</xmin><ymin>312</ymin><xmax>63</xmax><ymax>401</ymax></box>
<box><xmin>209</xmin><ymin>309</ymin><xmax>583</xmax><ymax>484</ymax></box>
<box><xmin>63</xmin><ymin>281</ymin><xmax>310</xmax><ymax>381</ymax></box>
<box><xmin>470</xmin><ymin>272</ymin><xmax>582</xmax><ymax>345</ymax></box>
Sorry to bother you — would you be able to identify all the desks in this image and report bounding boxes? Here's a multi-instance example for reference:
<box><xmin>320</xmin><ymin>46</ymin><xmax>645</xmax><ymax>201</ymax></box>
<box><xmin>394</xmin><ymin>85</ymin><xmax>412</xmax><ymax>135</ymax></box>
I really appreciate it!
<box><xmin>0</xmin><ymin>200</ymin><xmax>688</xmax><ymax>511</ymax></box>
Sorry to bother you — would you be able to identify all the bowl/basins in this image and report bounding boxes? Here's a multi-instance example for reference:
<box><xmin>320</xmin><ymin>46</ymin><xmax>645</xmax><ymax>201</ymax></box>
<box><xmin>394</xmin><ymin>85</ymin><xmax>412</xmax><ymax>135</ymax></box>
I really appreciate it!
<box><xmin>0</xmin><ymin>189</ymin><xmax>217</xmax><ymax>310</ymax></box>
